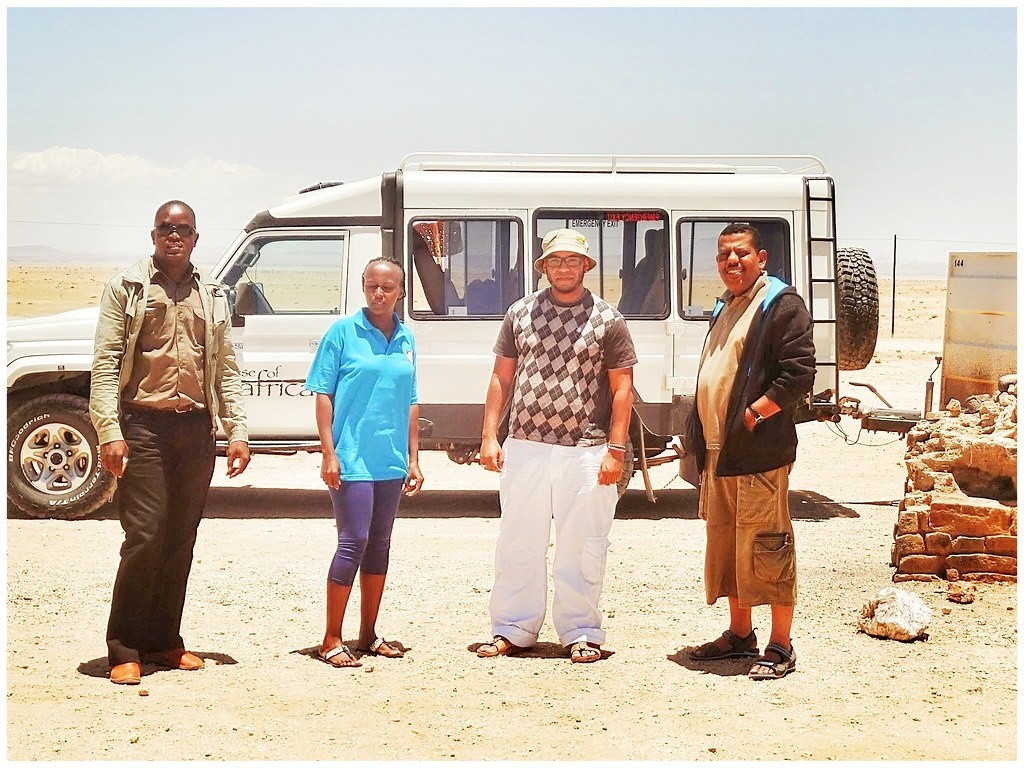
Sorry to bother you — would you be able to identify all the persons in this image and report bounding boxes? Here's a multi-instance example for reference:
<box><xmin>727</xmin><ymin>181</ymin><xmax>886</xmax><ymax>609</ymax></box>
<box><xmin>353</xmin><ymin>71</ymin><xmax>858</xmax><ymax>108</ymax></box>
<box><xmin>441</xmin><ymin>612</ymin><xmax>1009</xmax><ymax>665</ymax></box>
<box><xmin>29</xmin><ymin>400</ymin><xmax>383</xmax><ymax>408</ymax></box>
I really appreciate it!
<box><xmin>304</xmin><ymin>258</ymin><xmax>425</xmax><ymax>667</ymax></box>
<box><xmin>87</xmin><ymin>201</ymin><xmax>251</xmax><ymax>685</ymax></box>
<box><xmin>686</xmin><ymin>224</ymin><xmax>819</xmax><ymax>680</ymax></box>
<box><xmin>476</xmin><ymin>229</ymin><xmax>638</xmax><ymax>664</ymax></box>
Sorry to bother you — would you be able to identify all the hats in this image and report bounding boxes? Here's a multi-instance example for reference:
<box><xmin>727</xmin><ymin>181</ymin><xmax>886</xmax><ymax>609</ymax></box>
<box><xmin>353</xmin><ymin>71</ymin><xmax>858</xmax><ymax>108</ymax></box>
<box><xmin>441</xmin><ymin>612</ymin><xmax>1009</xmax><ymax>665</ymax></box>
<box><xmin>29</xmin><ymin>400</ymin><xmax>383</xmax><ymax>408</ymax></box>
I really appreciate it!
<box><xmin>534</xmin><ymin>228</ymin><xmax>596</xmax><ymax>275</ymax></box>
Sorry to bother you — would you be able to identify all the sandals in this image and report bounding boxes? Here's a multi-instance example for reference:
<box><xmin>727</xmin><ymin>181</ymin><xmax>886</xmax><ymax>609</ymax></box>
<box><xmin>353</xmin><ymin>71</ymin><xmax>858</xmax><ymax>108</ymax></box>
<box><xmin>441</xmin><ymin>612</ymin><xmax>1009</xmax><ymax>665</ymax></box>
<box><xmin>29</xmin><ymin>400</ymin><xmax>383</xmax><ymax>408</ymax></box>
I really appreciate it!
<box><xmin>687</xmin><ymin>627</ymin><xmax>759</xmax><ymax>660</ymax></box>
<box><xmin>748</xmin><ymin>637</ymin><xmax>796</xmax><ymax>680</ymax></box>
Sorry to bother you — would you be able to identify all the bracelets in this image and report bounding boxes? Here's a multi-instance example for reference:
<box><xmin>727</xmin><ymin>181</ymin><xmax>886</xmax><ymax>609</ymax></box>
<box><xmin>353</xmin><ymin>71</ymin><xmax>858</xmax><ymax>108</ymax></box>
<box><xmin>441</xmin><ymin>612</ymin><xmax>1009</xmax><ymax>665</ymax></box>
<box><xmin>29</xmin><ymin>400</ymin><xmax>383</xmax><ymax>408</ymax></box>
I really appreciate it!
<box><xmin>608</xmin><ymin>443</ymin><xmax>626</xmax><ymax>452</ymax></box>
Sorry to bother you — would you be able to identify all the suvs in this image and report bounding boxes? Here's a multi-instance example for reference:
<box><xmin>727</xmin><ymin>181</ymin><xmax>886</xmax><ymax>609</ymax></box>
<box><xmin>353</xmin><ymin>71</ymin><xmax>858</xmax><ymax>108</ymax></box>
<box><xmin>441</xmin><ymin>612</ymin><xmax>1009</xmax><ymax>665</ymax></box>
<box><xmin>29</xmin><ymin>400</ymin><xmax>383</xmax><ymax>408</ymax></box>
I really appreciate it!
<box><xmin>7</xmin><ymin>154</ymin><xmax>881</xmax><ymax>521</ymax></box>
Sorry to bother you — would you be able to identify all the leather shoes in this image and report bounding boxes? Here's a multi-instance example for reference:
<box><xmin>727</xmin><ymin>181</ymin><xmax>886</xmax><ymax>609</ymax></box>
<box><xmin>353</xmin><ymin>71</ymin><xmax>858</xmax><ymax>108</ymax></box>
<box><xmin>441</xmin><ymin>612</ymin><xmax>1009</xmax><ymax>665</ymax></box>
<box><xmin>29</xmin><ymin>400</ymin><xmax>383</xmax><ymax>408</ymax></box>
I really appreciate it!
<box><xmin>109</xmin><ymin>661</ymin><xmax>139</xmax><ymax>685</ymax></box>
<box><xmin>144</xmin><ymin>648</ymin><xmax>204</xmax><ymax>671</ymax></box>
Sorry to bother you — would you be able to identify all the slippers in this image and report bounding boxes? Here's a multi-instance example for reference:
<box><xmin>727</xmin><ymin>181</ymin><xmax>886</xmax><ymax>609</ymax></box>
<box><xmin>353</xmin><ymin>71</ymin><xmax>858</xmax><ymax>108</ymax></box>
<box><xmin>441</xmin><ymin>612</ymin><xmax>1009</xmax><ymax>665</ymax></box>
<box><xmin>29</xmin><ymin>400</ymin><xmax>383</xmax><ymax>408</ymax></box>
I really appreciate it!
<box><xmin>475</xmin><ymin>636</ymin><xmax>518</xmax><ymax>657</ymax></box>
<box><xmin>355</xmin><ymin>637</ymin><xmax>404</xmax><ymax>657</ymax></box>
<box><xmin>569</xmin><ymin>640</ymin><xmax>601</xmax><ymax>662</ymax></box>
<box><xmin>313</xmin><ymin>644</ymin><xmax>362</xmax><ymax>667</ymax></box>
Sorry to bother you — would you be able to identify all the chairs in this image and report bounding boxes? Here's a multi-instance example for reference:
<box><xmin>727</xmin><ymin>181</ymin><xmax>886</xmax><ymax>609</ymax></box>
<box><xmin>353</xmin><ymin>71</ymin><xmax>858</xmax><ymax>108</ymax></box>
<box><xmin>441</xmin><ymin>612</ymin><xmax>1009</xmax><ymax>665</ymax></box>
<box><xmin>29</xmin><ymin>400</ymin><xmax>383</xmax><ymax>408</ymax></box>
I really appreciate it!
<box><xmin>614</xmin><ymin>229</ymin><xmax>665</xmax><ymax>315</ymax></box>
<box><xmin>413</xmin><ymin>228</ymin><xmax>518</xmax><ymax>315</ymax></box>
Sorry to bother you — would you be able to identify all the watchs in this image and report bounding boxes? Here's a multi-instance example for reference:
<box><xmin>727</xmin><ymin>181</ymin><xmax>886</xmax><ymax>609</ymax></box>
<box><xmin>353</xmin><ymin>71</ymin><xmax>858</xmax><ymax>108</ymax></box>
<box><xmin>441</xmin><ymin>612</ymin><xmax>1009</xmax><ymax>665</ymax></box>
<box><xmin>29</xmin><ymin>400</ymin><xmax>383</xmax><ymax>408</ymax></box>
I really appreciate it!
<box><xmin>748</xmin><ymin>406</ymin><xmax>765</xmax><ymax>424</ymax></box>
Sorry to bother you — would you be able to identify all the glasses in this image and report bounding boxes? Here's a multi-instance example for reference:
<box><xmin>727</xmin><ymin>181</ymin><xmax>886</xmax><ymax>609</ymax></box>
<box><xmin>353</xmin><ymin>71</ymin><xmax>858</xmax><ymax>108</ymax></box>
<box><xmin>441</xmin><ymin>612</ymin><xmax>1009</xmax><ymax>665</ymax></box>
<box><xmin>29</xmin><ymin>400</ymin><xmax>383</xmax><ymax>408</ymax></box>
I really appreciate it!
<box><xmin>544</xmin><ymin>255</ymin><xmax>585</xmax><ymax>268</ymax></box>
<box><xmin>153</xmin><ymin>223</ymin><xmax>196</xmax><ymax>236</ymax></box>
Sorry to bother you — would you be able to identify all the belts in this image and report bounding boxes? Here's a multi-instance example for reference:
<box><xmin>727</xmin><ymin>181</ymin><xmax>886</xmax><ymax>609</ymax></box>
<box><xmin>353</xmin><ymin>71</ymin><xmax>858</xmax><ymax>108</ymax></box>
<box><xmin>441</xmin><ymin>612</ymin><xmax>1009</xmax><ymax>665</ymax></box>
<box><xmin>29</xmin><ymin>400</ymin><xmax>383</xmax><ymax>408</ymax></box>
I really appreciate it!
<box><xmin>156</xmin><ymin>403</ymin><xmax>209</xmax><ymax>413</ymax></box>
